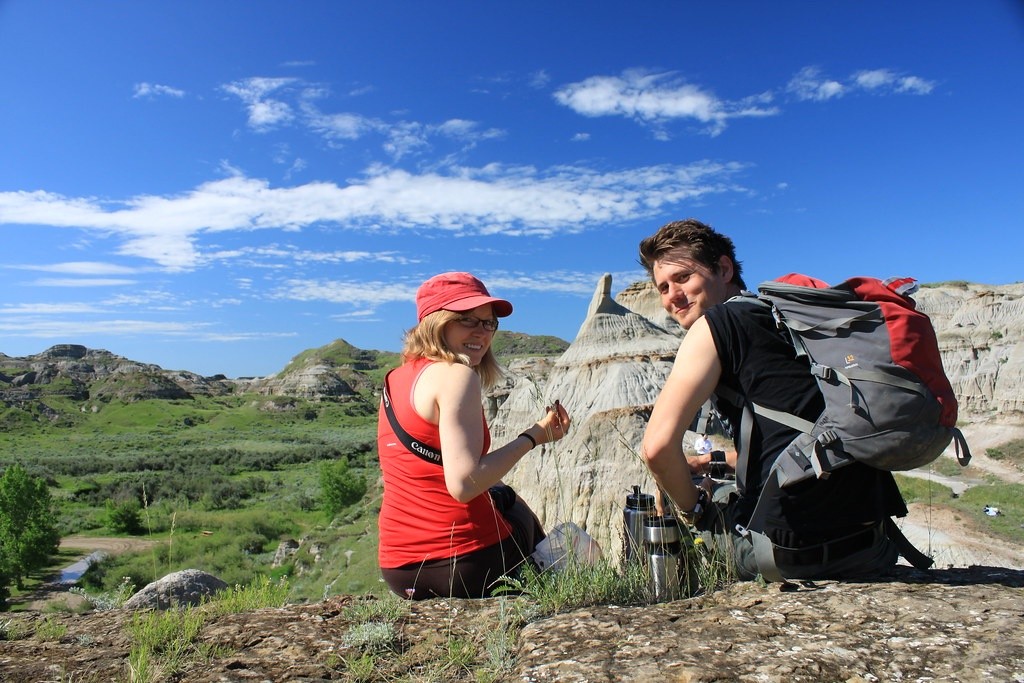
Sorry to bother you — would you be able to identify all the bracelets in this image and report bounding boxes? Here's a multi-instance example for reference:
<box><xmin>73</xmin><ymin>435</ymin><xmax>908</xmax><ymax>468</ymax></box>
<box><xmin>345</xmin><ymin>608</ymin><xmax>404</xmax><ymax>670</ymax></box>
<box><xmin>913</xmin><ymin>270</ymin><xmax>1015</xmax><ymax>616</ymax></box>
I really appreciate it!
<box><xmin>710</xmin><ymin>449</ymin><xmax>726</xmax><ymax>479</ymax></box>
<box><xmin>518</xmin><ymin>433</ymin><xmax>536</xmax><ymax>449</ymax></box>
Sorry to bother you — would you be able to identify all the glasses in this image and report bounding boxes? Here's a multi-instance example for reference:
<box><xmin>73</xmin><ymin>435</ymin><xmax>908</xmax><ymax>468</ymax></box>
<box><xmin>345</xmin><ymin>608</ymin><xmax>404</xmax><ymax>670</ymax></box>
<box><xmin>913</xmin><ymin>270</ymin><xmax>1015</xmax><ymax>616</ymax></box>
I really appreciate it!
<box><xmin>454</xmin><ymin>314</ymin><xmax>499</xmax><ymax>331</ymax></box>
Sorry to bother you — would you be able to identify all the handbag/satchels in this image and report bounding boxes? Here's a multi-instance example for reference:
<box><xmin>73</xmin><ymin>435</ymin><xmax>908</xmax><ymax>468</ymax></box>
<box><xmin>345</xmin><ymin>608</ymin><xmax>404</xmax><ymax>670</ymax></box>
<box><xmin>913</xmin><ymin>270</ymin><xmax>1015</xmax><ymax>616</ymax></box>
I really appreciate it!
<box><xmin>488</xmin><ymin>485</ymin><xmax>546</xmax><ymax>569</ymax></box>
<box><xmin>531</xmin><ymin>522</ymin><xmax>605</xmax><ymax>572</ymax></box>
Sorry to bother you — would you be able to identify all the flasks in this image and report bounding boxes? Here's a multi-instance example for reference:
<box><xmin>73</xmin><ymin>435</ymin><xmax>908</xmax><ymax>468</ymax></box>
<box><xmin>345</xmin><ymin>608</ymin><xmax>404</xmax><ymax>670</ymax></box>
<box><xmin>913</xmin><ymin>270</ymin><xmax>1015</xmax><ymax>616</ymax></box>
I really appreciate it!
<box><xmin>643</xmin><ymin>515</ymin><xmax>682</xmax><ymax>605</ymax></box>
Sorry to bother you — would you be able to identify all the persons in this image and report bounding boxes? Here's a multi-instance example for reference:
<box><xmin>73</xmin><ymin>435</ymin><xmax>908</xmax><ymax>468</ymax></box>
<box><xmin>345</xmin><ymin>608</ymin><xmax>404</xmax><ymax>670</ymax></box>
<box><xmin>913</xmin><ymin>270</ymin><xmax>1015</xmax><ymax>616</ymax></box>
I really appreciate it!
<box><xmin>640</xmin><ymin>219</ymin><xmax>903</xmax><ymax>581</ymax></box>
<box><xmin>377</xmin><ymin>272</ymin><xmax>571</xmax><ymax>600</ymax></box>
<box><xmin>694</xmin><ymin>434</ymin><xmax>712</xmax><ymax>456</ymax></box>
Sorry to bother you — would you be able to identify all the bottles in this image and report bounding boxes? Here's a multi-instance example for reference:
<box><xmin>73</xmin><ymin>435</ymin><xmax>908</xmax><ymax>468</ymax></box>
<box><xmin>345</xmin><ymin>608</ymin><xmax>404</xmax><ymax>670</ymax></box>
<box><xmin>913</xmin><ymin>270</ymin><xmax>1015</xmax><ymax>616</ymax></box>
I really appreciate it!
<box><xmin>623</xmin><ymin>485</ymin><xmax>658</xmax><ymax>562</ymax></box>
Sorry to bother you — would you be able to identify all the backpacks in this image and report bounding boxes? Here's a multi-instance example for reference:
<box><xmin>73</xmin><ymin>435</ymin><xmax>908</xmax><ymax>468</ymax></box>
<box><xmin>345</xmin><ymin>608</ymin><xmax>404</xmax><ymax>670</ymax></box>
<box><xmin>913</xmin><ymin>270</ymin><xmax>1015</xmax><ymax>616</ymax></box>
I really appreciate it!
<box><xmin>722</xmin><ymin>273</ymin><xmax>971</xmax><ymax>584</ymax></box>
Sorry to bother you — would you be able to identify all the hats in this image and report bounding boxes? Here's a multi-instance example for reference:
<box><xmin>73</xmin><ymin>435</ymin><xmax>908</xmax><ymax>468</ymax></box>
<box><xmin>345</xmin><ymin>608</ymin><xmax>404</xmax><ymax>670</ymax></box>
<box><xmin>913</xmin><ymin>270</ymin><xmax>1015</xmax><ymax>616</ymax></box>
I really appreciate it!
<box><xmin>416</xmin><ymin>272</ymin><xmax>512</xmax><ymax>323</ymax></box>
